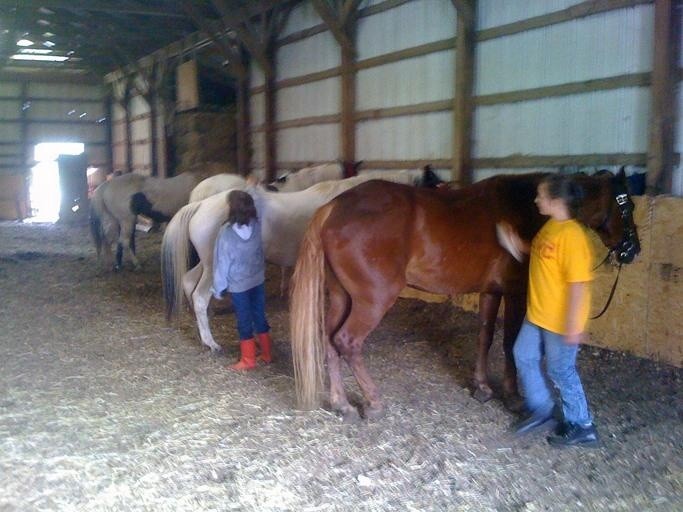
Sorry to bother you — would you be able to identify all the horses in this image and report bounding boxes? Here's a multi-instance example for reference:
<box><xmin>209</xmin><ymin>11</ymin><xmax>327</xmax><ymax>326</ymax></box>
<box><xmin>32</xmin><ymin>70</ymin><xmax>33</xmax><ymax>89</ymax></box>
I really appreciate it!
<box><xmin>183</xmin><ymin>157</ymin><xmax>364</xmax><ymax>204</ymax></box>
<box><xmin>90</xmin><ymin>147</ymin><xmax>244</xmax><ymax>272</ymax></box>
<box><xmin>158</xmin><ymin>160</ymin><xmax>445</xmax><ymax>356</ymax></box>
<box><xmin>285</xmin><ymin>160</ymin><xmax>642</xmax><ymax>427</ymax></box>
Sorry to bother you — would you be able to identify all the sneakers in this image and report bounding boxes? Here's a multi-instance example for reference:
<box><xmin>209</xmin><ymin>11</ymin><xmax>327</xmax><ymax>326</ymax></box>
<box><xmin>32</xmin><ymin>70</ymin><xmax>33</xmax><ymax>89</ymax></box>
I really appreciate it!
<box><xmin>513</xmin><ymin>407</ymin><xmax>600</xmax><ymax>449</ymax></box>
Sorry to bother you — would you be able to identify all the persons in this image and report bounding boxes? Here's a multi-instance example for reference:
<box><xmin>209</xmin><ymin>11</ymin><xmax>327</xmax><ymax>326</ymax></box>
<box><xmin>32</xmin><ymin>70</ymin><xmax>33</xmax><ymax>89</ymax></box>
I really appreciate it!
<box><xmin>495</xmin><ymin>172</ymin><xmax>600</xmax><ymax>446</ymax></box>
<box><xmin>113</xmin><ymin>169</ymin><xmax>136</xmax><ymax>271</ymax></box>
<box><xmin>210</xmin><ymin>174</ymin><xmax>274</xmax><ymax>370</ymax></box>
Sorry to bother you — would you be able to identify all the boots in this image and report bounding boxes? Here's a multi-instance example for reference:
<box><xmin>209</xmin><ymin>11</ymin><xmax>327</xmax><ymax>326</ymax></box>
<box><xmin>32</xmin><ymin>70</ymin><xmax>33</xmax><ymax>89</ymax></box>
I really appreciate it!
<box><xmin>255</xmin><ymin>331</ymin><xmax>272</xmax><ymax>364</ymax></box>
<box><xmin>227</xmin><ymin>337</ymin><xmax>257</xmax><ymax>370</ymax></box>
